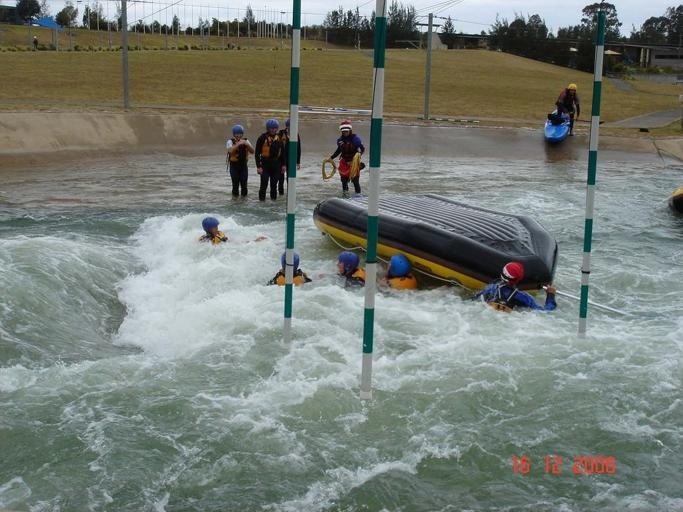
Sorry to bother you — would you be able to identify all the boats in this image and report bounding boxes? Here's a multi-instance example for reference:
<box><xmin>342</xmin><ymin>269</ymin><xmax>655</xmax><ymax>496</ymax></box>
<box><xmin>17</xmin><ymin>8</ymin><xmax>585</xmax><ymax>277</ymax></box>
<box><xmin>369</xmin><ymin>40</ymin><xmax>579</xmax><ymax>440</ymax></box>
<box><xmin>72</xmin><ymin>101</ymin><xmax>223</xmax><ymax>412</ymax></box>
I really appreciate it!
<box><xmin>312</xmin><ymin>192</ymin><xmax>557</xmax><ymax>292</ymax></box>
<box><xmin>543</xmin><ymin>108</ymin><xmax>570</xmax><ymax>143</ymax></box>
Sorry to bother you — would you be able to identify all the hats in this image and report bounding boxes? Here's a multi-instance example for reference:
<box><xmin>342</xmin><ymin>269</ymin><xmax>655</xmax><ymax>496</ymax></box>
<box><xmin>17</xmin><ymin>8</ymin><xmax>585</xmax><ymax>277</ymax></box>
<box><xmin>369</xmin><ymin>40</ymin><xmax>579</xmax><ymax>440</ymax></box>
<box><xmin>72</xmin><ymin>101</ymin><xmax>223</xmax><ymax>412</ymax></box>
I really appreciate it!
<box><xmin>501</xmin><ymin>262</ymin><xmax>524</xmax><ymax>285</ymax></box>
<box><xmin>339</xmin><ymin>121</ymin><xmax>352</xmax><ymax>131</ymax></box>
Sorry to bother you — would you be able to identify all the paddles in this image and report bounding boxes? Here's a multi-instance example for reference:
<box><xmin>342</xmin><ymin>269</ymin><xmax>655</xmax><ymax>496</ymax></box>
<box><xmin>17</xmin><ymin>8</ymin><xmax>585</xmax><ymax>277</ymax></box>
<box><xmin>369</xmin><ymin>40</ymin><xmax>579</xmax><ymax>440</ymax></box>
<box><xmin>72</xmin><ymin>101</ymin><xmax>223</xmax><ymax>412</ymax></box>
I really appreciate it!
<box><xmin>574</xmin><ymin>117</ymin><xmax>606</xmax><ymax>128</ymax></box>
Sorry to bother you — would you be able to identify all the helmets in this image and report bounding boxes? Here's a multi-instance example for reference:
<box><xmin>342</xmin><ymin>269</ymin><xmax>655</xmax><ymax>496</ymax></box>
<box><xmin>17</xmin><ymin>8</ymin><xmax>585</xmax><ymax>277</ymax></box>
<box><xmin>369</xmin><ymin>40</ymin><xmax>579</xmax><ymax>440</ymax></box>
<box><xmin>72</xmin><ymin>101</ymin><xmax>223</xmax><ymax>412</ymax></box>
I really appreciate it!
<box><xmin>339</xmin><ymin>251</ymin><xmax>359</xmax><ymax>271</ymax></box>
<box><xmin>202</xmin><ymin>218</ymin><xmax>219</xmax><ymax>231</ymax></box>
<box><xmin>266</xmin><ymin>119</ymin><xmax>279</xmax><ymax>134</ymax></box>
<box><xmin>282</xmin><ymin>251</ymin><xmax>299</xmax><ymax>270</ymax></box>
<box><xmin>233</xmin><ymin>125</ymin><xmax>243</xmax><ymax>134</ymax></box>
<box><xmin>568</xmin><ymin>84</ymin><xmax>576</xmax><ymax>90</ymax></box>
<box><xmin>388</xmin><ymin>254</ymin><xmax>411</xmax><ymax>277</ymax></box>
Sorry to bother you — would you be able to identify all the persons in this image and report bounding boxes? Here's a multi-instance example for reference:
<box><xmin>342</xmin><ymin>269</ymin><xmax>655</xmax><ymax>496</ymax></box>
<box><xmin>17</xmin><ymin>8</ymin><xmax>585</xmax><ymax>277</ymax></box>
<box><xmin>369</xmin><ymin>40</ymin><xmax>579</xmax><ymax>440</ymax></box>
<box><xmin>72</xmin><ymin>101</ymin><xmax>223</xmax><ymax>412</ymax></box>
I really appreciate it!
<box><xmin>254</xmin><ymin>118</ymin><xmax>287</xmax><ymax>203</ymax></box>
<box><xmin>279</xmin><ymin>117</ymin><xmax>302</xmax><ymax>196</ymax></box>
<box><xmin>265</xmin><ymin>247</ymin><xmax>314</xmax><ymax>285</ymax></box>
<box><xmin>471</xmin><ymin>261</ymin><xmax>559</xmax><ymax>310</ymax></box>
<box><xmin>225</xmin><ymin>123</ymin><xmax>255</xmax><ymax>203</ymax></box>
<box><xmin>318</xmin><ymin>251</ymin><xmax>366</xmax><ymax>283</ymax></box>
<box><xmin>194</xmin><ymin>214</ymin><xmax>270</xmax><ymax>246</ymax></box>
<box><xmin>32</xmin><ymin>35</ymin><xmax>42</xmax><ymax>53</ymax></box>
<box><xmin>328</xmin><ymin>119</ymin><xmax>367</xmax><ymax>194</ymax></box>
<box><xmin>382</xmin><ymin>250</ymin><xmax>419</xmax><ymax>293</ymax></box>
<box><xmin>553</xmin><ymin>82</ymin><xmax>581</xmax><ymax>136</ymax></box>
